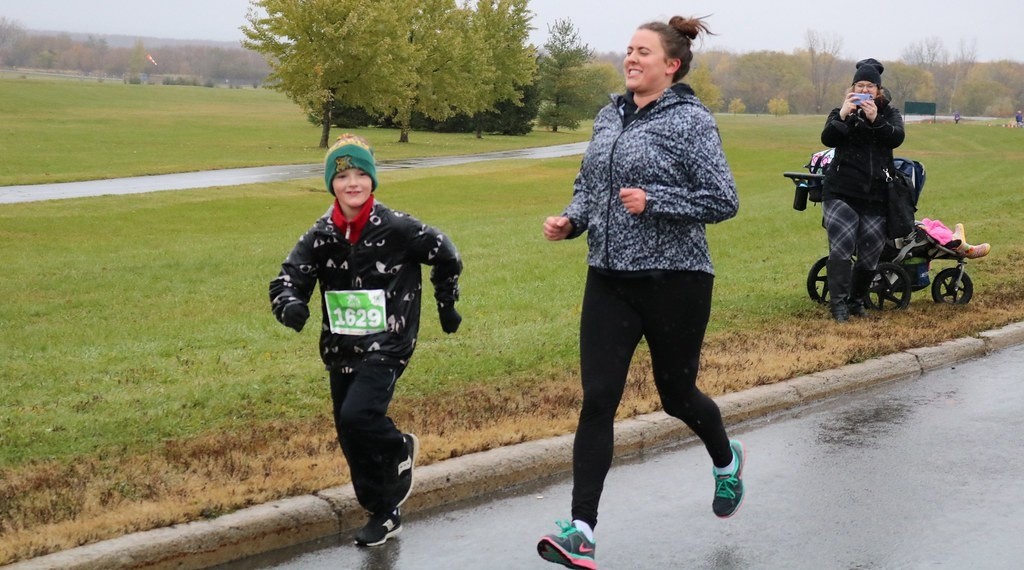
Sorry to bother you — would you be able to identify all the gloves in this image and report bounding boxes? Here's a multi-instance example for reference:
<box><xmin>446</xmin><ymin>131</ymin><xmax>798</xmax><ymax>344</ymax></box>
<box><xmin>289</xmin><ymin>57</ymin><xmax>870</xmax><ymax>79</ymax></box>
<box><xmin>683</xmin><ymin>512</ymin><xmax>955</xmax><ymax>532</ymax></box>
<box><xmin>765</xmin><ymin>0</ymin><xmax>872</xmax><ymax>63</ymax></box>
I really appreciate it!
<box><xmin>438</xmin><ymin>309</ymin><xmax>461</xmax><ymax>333</ymax></box>
<box><xmin>284</xmin><ymin>304</ymin><xmax>310</xmax><ymax>333</ymax></box>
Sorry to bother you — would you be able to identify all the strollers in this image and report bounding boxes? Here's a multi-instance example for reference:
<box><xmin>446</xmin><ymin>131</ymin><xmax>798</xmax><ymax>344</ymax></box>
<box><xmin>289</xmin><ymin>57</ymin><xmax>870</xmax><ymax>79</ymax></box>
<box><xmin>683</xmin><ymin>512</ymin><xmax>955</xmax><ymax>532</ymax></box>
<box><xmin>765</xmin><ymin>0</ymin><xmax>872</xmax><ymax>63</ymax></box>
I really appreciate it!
<box><xmin>784</xmin><ymin>148</ymin><xmax>973</xmax><ymax>312</ymax></box>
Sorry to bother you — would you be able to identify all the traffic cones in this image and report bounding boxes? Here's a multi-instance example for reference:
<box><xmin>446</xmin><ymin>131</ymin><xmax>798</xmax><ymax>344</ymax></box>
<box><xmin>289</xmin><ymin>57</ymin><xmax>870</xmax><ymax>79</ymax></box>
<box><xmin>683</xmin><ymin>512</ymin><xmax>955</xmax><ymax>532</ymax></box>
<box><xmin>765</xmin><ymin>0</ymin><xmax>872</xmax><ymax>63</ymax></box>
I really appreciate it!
<box><xmin>1002</xmin><ymin>124</ymin><xmax>1018</xmax><ymax>128</ymax></box>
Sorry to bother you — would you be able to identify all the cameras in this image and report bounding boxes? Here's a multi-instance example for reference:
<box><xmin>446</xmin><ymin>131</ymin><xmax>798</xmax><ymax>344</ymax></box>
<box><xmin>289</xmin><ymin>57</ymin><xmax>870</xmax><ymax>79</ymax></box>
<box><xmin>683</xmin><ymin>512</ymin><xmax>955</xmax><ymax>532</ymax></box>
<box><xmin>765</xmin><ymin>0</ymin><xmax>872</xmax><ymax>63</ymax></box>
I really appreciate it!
<box><xmin>850</xmin><ymin>93</ymin><xmax>875</xmax><ymax>105</ymax></box>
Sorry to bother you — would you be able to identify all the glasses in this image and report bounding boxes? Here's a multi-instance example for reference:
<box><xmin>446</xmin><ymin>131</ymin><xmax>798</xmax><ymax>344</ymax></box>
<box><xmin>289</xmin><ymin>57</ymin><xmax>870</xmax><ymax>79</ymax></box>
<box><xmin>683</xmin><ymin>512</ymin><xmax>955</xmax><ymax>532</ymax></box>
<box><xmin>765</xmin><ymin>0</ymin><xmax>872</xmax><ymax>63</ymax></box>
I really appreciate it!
<box><xmin>853</xmin><ymin>82</ymin><xmax>876</xmax><ymax>89</ymax></box>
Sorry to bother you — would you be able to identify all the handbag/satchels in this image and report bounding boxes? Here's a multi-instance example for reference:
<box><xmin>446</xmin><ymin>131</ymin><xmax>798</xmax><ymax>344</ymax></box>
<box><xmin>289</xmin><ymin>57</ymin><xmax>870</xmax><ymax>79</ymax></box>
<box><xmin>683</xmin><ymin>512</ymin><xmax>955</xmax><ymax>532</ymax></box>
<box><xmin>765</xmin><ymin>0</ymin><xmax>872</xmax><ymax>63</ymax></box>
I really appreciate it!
<box><xmin>883</xmin><ymin>169</ymin><xmax>917</xmax><ymax>238</ymax></box>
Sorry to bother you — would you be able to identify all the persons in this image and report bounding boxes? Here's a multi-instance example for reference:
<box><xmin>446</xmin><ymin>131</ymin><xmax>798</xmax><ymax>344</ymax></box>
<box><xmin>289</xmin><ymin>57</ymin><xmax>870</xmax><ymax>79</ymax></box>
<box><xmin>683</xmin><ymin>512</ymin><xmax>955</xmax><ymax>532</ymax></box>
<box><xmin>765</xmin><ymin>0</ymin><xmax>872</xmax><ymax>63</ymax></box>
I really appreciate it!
<box><xmin>821</xmin><ymin>58</ymin><xmax>905</xmax><ymax>323</ymax></box>
<box><xmin>1015</xmin><ymin>111</ymin><xmax>1022</xmax><ymax>122</ymax></box>
<box><xmin>921</xmin><ymin>218</ymin><xmax>991</xmax><ymax>259</ymax></box>
<box><xmin>955</xmin><ymin>111</ymin><xmax>960</xmax><ymax>124</ymax></box>
<box><xmin>536</xmin><ymin>17</ymin><xmax>747</xmax><ymax>570</ymax></box>
<box><xmin>269</xmin><ymin>133</ymin><xmax>463</xmax><ymax>546</ymax></box>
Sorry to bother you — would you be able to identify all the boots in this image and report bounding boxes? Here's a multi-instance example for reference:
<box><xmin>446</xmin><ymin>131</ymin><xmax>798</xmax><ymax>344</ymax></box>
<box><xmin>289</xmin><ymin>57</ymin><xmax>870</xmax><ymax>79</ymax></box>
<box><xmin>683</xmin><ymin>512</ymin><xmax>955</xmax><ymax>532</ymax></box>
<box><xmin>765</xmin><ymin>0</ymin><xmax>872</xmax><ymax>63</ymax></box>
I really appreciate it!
<box><xmin>952</xmin><ymin>223</ymin><xmax>966</xmax><ymax>243</ymax></box>
<box><xmin>851</xmin><ymin>267</ymin><xmax>869</xmax><ymax>317</ymax></box>
<box><xmin>952</xmin><ymin>241</ymin><xmax>990</xmax><ymax>259</ymax></box>
<box><xmin>826</xmin><ymin>259</ymin><xmax>852</xmax><ymax>321</ymax></box>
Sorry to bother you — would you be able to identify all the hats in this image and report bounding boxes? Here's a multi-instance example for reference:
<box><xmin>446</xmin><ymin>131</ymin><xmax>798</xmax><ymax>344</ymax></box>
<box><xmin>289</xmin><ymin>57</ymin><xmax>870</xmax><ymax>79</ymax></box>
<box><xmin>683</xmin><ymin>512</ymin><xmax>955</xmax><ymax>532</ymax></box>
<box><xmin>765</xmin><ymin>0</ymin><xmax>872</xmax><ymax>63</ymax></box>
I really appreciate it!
<box><xmin>851</xmin><ymin>58</ymin><xmax>883</xmax><ymax>90</ymax></box>
<box><xmin>324</xmin><ymin>133</ymin><xmax>378</xmax><ymax>197</ymax></box>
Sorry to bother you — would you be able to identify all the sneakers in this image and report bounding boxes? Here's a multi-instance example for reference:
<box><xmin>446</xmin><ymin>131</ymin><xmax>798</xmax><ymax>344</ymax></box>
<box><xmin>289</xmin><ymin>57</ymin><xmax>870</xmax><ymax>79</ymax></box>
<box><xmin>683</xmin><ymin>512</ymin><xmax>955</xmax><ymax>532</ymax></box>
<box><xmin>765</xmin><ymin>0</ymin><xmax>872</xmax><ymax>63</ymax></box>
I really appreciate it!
<box><xmin>387</xmin><ymin>433</ymin><xmax>418</xmax><ymax>508</ymax></box>
<box><xmin>537</xmin><ymin>519</ymin><xmax>597</xmax><ymax>570</ymax></box>
<box><xmin>712</xmin><ymin>437</ymin><xmax>746</xmax><ymax>518</ymax></box>
<box><xmin>353</xmin><ymin>512</ymin><xmax>403</xmax><ymax>547</ymax></box>
